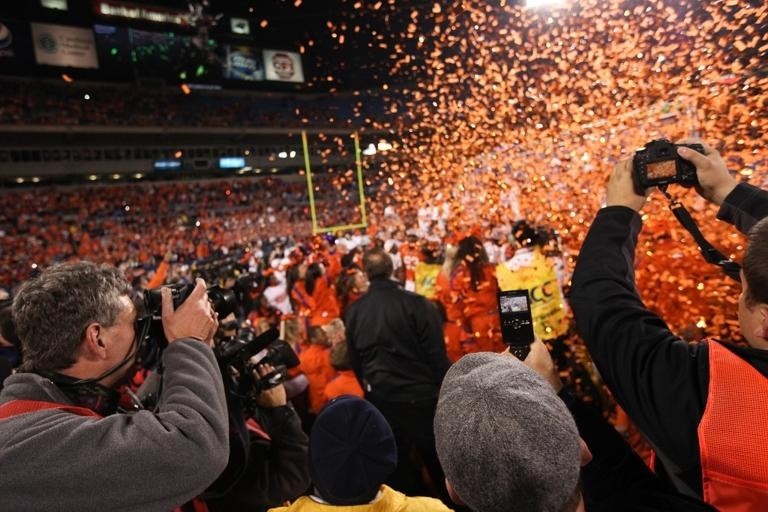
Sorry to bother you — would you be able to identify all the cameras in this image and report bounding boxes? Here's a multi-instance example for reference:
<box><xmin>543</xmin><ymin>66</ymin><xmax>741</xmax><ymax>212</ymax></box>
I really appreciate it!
<box><xmin>631</xmin><ymin>139</ymin><xmax>705</xmax><ymax>195</ymax></box>
<box><xmin>135</xmin><ymin>281</ymin><xmax>238</xmax><ymax>372</ymax></box>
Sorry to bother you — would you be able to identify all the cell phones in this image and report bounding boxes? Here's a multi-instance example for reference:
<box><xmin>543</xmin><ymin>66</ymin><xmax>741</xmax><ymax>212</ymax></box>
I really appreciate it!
<box><xmin>496</xmin><ymin>289</ymin><xmax>535</xmax><ymax>360</ymax></box>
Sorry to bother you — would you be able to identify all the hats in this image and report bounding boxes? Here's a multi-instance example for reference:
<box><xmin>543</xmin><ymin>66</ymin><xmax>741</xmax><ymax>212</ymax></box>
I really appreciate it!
<box><xmin>433</xmin><ymin>351</ymin><xmax>581</xmax><ymax>512</ymax></box>
<box><xmin>310</xmin><ymin>393</ymin><xmax>398</xmax><ymax>502</ymax></box>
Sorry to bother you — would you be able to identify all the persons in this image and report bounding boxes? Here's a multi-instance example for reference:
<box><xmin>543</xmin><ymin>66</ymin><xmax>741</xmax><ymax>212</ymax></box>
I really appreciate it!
<box><xmin>130</xmin><ymin>25</ymin><xmax>294</xmax><ymax>83</ymax></box>
<box><xmin>0</xmin><ymin>88</ymin><xmax>768</xmax><ymax>511</ymax></box>
